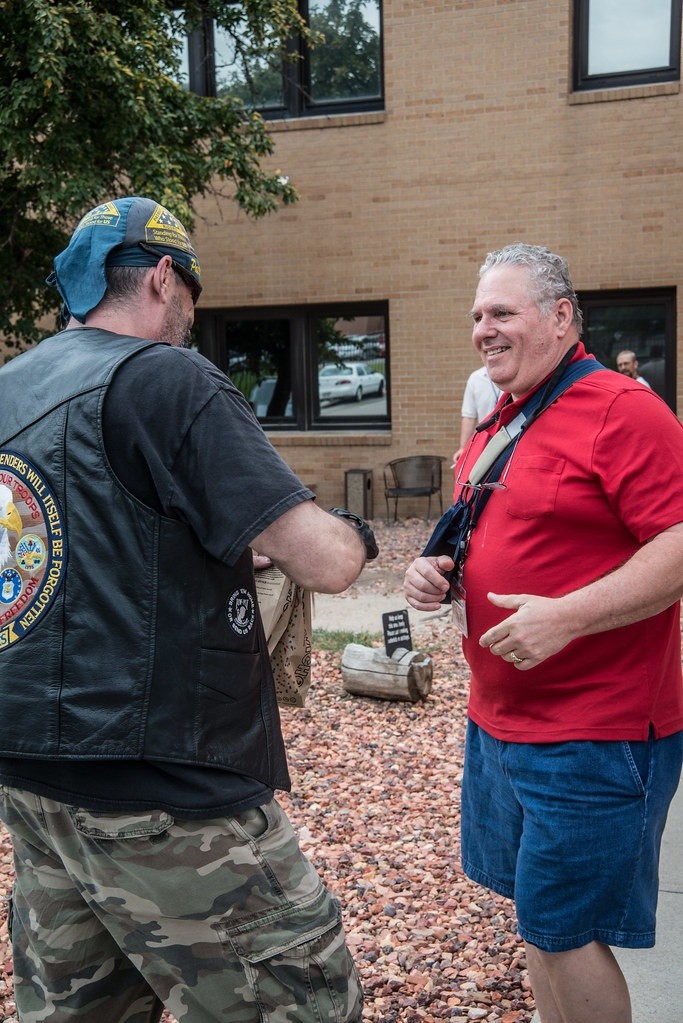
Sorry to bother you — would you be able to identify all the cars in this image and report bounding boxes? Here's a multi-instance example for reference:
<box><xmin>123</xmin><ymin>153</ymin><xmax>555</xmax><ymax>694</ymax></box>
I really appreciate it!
<box><xmin>315</xmin><ymin>330</ymin><xmax>388</xmax><ymax>361</ymax></box>
<box><xmin>249</xmin><ymin>378</ymin><xmax>295</xmax><ymax>420</ymax></box>
<box><xmin>317</xmin><ymin>360</ymin><xmax>386</xmax><ymax>403</ymax></box>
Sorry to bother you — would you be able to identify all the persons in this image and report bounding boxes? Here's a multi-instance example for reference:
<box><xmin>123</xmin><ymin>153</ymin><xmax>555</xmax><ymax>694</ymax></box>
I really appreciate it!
<box><xmin>0</xmin><ymin>198</ymin><xmax>379</xmax><ymax>1023</ymax></box>
<box><xmin>454</xmin><ymin>366</ymin><xmax>504</xmax><ymax>465</ymax></box>
<box><xmin>617</xmin><ymin>349</ymin><xmax>650</xmax><ymax>390</ymax></box>
<box><xmin>403</xmin><ymin>242</ymin><xmax>683</xmax><ymax>1023</ymax></box>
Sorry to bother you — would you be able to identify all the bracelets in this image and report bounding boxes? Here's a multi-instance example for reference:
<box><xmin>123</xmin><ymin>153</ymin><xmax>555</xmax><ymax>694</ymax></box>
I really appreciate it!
<box><xmin>332</xmin><ymin>506</ymin><xmax>379</xmax><ymax>559</ymax></box>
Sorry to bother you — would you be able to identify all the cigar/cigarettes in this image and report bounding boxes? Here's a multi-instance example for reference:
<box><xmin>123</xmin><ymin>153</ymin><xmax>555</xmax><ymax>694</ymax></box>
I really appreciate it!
<box><xmin>451</xmin><ymin>464</ymin><xmax>456</xmax><ymax>469</ymax></box>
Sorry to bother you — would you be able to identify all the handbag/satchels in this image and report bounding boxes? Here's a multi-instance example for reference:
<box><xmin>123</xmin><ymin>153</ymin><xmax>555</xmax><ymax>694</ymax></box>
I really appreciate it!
<box><xmin>421</xmin><ymin>502</ymin><xmax>472</xmax><ymax>605</ymax></box>
<box><xmin>253</xmin><ymin>559</ymin><xmax>311</xmax><ymax>708</ymax></box>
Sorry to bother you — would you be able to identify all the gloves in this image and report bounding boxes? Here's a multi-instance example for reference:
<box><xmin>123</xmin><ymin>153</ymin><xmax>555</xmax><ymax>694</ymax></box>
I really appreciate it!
<box><xmin>329</xmin><ymin>508</ymin><xmax>380</xmax><ymax>563</ymax></box>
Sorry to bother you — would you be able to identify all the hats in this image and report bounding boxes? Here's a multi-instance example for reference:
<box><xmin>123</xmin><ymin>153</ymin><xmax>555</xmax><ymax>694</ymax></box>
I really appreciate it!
<box><xmin>45</xmin><ymin>196</ymin><xmax>203</xmax><ymax>325</ymax></box>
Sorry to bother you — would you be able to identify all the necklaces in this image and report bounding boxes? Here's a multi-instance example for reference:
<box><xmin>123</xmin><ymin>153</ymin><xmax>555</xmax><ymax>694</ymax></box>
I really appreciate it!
<box><xmin>491</xmin><ymin>383</ymin><xmax>500</xmax><ymax>405</ymax></box>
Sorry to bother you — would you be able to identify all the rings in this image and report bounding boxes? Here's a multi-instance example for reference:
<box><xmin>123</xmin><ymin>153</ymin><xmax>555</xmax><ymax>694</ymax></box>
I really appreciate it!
<box><xmin>510</xmin><ymin>650</ymin><xmax>522</xmax><ymax>663</ymax></box>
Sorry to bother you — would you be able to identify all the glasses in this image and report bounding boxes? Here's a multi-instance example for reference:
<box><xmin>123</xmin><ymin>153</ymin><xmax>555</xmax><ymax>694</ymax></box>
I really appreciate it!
<box><xmin>456</xmin><ymin>429</ymin><xmax>521</xmax><ymax>493</ymax></box>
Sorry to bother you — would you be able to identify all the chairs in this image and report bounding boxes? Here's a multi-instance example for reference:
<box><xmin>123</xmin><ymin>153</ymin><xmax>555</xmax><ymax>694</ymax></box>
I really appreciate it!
<box><xmin>384</xmin><ymin>455</ymin><xmax>446</xmax><ymax>526</ymax></box>
